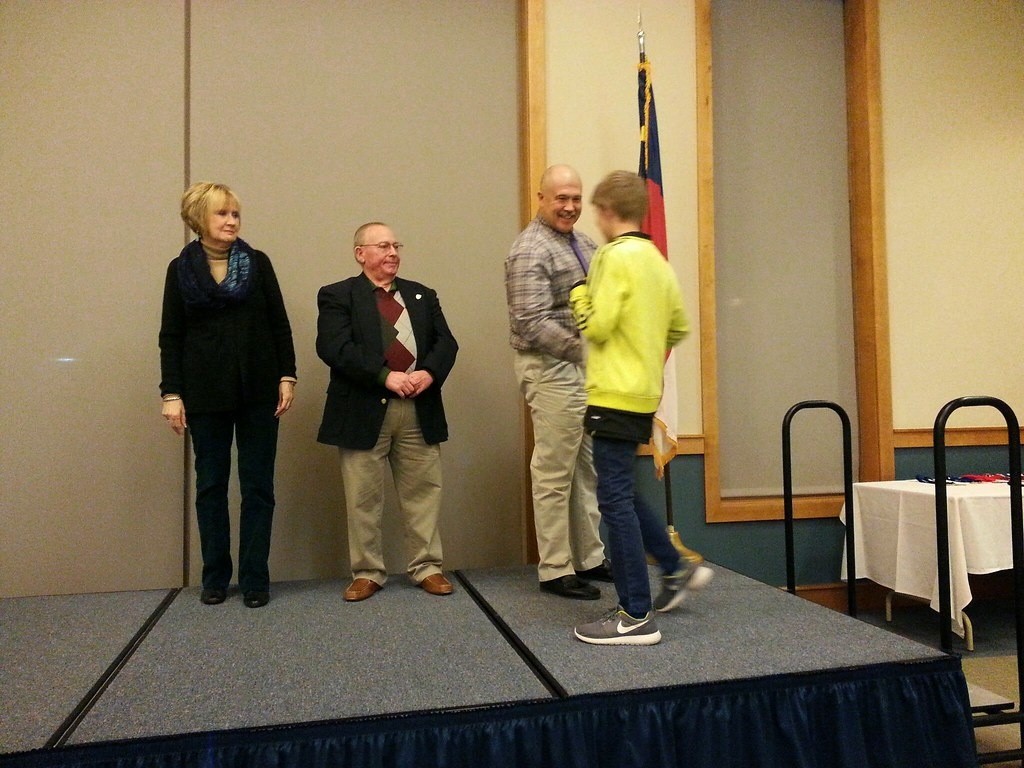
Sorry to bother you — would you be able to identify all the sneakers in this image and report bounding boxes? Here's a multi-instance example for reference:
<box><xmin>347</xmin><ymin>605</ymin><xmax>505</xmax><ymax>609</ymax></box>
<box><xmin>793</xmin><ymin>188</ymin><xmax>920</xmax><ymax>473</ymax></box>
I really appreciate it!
<box><xmin>652</xmin><ymin>557</ymin><xmax>714</xmax><ymax>611</ymax></box>
<box><xmin>574</xmin><ymin>602</ymin><xmax>662</xmax><ymax>645</ymax></box>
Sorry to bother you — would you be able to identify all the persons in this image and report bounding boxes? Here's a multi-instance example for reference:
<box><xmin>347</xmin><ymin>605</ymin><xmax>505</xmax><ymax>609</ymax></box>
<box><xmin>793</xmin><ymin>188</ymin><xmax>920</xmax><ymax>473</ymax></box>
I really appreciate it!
<box><xmin>315</xmin><ymin>222</ymin><xmax>459</xmax><ymax>600</ymax></box>
<box><xmin>570</xmin><ymin>170</ymin><xmax>713</xmax><ymax>645</ymax></box>
<box><xmin>158</xmin><ymin>183</ymin><xmax>297</xmax><ymax>607</ymax></box>
<box><xmin>504</xmin><ymin>165</ymin><xmax>615</xmax><ymax>600</ymax></box>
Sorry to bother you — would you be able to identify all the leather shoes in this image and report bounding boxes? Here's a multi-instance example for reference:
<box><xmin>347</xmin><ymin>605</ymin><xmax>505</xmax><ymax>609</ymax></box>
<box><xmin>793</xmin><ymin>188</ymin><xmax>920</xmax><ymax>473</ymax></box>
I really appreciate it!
<box><xmin>345</xmin><ymin>578</ymin><xmax>379</xmax><ymax>600</ymax></box>
<box><xmin>575</xmin><ymin>558</ymin><xmax>616</xmax><ymax>584</ymax></box>
<box><xmin>539</xmin><ymin>573</ymin><xmax>601</xmax><ymax>600</ymax></box>
<box><xmin>419</xmin><ymin>573</ymin><xmax>453</xmax><ymax>594</ymax></box>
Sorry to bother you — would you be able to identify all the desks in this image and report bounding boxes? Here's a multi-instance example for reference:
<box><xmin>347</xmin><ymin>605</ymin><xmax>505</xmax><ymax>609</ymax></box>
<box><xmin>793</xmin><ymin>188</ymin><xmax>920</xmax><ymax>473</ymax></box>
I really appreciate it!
<box><xmin>839</xmin><ymin>479</ymin><xmax>1024</xmax><ymax>652</ymax></box>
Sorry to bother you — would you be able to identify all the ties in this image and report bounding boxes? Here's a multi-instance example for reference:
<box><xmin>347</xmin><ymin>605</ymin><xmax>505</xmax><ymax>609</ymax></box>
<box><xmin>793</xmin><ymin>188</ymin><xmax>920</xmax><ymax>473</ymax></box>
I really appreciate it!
<box><xmin>569</xmin><ymin>234</ymin><xmax>589</xmax><ymax>274</ymax></box>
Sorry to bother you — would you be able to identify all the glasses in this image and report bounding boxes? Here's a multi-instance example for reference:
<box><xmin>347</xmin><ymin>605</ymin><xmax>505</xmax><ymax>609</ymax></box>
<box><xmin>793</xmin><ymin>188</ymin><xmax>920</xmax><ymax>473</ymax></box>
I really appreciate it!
<box><xmin>354</xmin><ymin>241</ymin><xmax>404</xmax><ymax>252</ymax></box>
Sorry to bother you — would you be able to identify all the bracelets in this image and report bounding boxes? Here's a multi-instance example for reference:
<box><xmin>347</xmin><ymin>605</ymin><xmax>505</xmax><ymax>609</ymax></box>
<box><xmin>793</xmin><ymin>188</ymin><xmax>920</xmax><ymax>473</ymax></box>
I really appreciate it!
<box><xmin>162</xmin><ymin>397</ymin><xmax>179</xmax><ymax>401</ymax></box>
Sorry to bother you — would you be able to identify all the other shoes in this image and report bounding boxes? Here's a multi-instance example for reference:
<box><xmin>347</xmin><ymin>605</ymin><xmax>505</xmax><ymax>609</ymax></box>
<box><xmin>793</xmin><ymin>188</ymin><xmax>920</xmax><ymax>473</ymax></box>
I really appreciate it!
<box><xmin>201</xmin><ymin>586</ymin><xmax>226</xmax><ymax>604</ymax></box>
<box><xmin>244</xmin><ymin>590</ymin><xmax>269</xmax><ymax>607</ymax></box>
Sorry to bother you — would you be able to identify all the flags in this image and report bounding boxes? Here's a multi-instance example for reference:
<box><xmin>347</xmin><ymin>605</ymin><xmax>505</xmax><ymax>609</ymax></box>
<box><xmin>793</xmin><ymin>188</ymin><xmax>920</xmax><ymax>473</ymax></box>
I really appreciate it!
<box><xmin>638</xmin><ymin>58</ymin><xmax>679</xmax><ymax>479</ymax></box>
